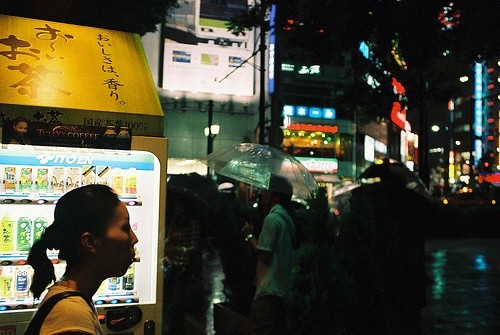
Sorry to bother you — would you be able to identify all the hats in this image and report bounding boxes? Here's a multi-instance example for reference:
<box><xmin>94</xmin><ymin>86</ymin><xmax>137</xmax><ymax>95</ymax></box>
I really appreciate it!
<box><xmin>385</xmin><ymin>167</ymin><xmax>409</xmax><ymax>187</ymax></box>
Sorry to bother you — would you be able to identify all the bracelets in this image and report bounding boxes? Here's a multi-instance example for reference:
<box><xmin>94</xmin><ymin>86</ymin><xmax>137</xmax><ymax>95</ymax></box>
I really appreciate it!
<box><xmin>244</xmin><ymin>233</ymin><xmax>253</xmax><ymax>242</ymax></box>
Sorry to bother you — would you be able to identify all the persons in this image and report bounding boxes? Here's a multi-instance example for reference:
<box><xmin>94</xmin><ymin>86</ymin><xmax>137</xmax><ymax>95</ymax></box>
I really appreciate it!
<box><xmin>239</xmin><ymin>175</ymin><xmax>296</xmax><ymax>335</ymax></box>
<box><xmin>22</xmin><ymin>184</ymin><xmax>139</xmax><ymax>335</ymax></box>
<box><xmin>164</xmin><ymin>169</ymin><xmax>453</xmax><ymax>335</ymax></box>
<box><xmin>8</xmin><ymin>116</ymin><xmax>31</xmax><ymax>145</ymax></box>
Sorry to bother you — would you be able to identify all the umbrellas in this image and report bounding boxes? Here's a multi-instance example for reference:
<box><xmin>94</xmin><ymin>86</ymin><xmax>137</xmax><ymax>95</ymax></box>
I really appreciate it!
<box><xmin>167</xmin><ymin>171</ymin><xmax>228</xmax><ymax>216</ymax></box>
<box><xmin>198</xmin><ymin>143</ymin><xmax>320</xmax><ymax>227</ymax></box>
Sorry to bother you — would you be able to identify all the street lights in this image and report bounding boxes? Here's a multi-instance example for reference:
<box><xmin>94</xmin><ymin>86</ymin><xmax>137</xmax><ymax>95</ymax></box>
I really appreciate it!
<box><xmin>204</xmin><ymin>101</ymin><xmax>220</xmax><ymax>176</ymax></box>
